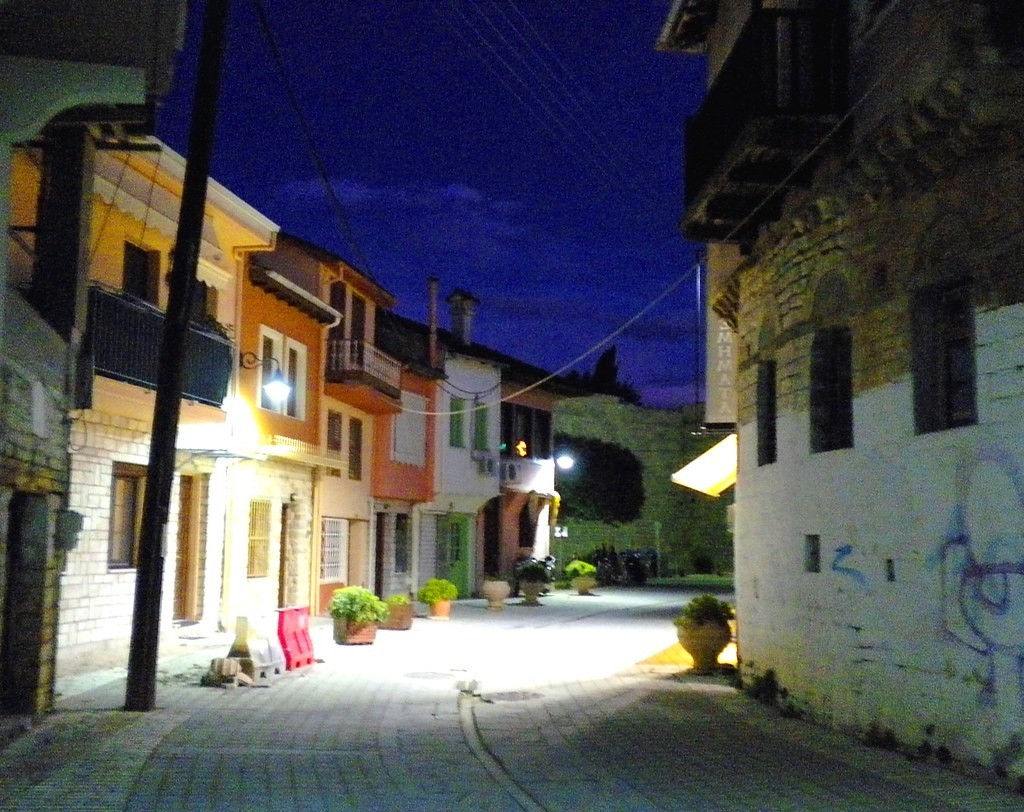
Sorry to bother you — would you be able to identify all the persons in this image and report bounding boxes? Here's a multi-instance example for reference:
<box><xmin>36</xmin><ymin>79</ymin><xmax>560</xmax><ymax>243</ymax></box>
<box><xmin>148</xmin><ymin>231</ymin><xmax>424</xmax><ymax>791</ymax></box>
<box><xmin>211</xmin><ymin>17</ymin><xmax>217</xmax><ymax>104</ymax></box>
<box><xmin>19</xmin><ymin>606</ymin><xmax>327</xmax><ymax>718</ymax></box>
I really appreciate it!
<box><xmin>593</xmin><ymin>543</ymin><xmax>621</xmax><ymax>580</ymax></box>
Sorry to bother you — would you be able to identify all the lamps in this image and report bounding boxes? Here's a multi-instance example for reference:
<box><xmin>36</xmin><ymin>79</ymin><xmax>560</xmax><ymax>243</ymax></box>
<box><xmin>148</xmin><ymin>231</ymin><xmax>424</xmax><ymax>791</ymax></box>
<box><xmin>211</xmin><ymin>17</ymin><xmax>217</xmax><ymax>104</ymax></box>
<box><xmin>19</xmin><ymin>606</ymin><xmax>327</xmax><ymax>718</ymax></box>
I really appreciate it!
<box><xmin>239</xmin><ymin>351</ymin><xmax>294</xmax><ymax>411</ymax></box>
<box><xmin>552</xmin><ymin>443</ymin><xmax>574</xmax><ymax>470</ymax></box>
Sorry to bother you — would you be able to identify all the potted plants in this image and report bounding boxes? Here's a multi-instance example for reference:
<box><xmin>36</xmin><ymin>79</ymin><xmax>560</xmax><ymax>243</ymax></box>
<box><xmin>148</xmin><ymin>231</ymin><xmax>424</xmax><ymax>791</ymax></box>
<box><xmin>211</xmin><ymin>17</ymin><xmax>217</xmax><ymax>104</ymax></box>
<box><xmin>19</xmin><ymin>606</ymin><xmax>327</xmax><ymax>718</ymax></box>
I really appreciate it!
<box><xmin>480</xmin><ymin>573</ymin><xmax>518</xmax><ymax>610</ymax></box>
<box><xmin>564</xmin><ymin>560</ymin><xmax>602</xmax><ymax>595</ymax></box>
<box><xmin>673</xmin><ymin>593</ymin><xmax>736</xmax><ymax>674</ymax></box>
<box><xmin>327</xmin><ymin>585</ymin><xmax>391</xmax><ymax>646</ymax></box>
<box><xmin>377</xmin><ymin>594</ymin><xmax>413</xmax><ymax>631</ymax></box>
<box><xmin>419</xmin><ymin>577</ymin><xmax>460</xmax><ymax>619</ymax></box>
<box><xmin>514</xmin><ymin>563</ymin><xmax>549</xmax><ymax>602</ymax></box>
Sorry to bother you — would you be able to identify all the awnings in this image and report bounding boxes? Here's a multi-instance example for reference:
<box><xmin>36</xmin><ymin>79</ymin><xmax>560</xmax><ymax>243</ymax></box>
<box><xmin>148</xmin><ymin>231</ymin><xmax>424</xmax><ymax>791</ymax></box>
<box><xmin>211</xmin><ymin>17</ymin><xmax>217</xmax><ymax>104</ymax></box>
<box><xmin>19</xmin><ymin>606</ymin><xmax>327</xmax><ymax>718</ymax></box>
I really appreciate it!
<box><xmin>670</xmin><ymin>434</ymin><xmax>737</xmax><ymax>498</ymax></box>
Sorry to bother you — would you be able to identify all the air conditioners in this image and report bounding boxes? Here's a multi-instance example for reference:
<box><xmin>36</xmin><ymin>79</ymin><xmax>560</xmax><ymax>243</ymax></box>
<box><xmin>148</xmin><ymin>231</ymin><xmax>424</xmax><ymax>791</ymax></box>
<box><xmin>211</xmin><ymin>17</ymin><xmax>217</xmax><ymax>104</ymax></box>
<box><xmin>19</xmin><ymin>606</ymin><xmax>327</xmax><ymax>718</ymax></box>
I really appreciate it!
<box><xmin>481</xmin><ymin>458</ymin><xmax>498</xmax><ymax>475</ymax></box>
<box><xmin>502</xmin><ymin>462</ymin><xmax>523</xmax><ymax>485</ymax></box>
